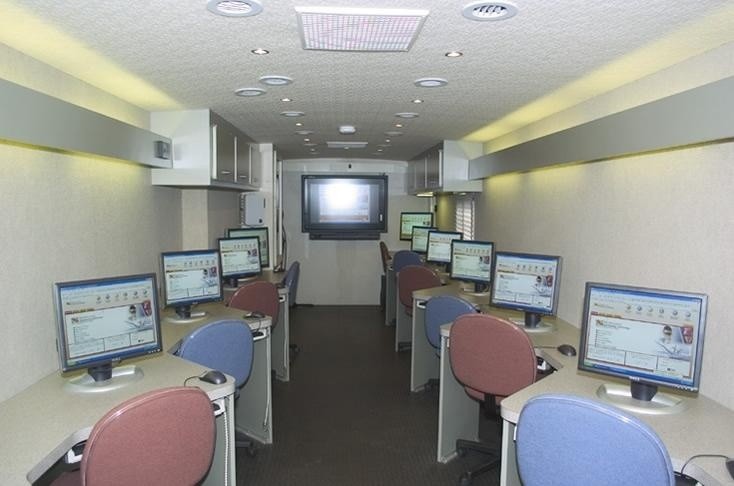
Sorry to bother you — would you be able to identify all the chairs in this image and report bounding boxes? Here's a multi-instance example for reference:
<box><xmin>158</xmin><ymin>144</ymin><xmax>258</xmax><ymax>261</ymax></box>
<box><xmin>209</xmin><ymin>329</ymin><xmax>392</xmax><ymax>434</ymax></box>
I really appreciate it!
<box><xmin>174</xmin><ymin>319</ymin><xmax>260</xmax><ymax>456</ymax></box>
<box><xmin>450</xmin><ymin>313</ymin><xmax>537</xmax><ymax>485</ymax></box>
<box><xmin>281</xmin><ymin>260</ymin><xmax>300</xmax><ymax>353</ymax></box>
<box><xmin>424</xmin><ymin>295</ymin><xmax>479</xmax><ymax>388</ymax></box>
<box><xmin>227</xmin><ymin>280</ymin><xmax>280</xmax><ymax>382</ymax></box>
<box><xmin>397</xmin><ymin>264</ymin><xmax>442</xmax><ymax>350</ymax></box>
<box><xmin>79</xmin><ymin>385</ymin><xmax>217</xmax><ymax>485</ymax></box>
<box><xmin>389</xmin><ymin>251</ymin><xmax>425</xmax><ymax>327</ymax></box>
<box><xmin>380</xmin><ymin>241</ymin><xmax>391</xmax><ymax>311</ymax></box>
<box><xmin>515</xmin><ymin>395</ymin><xmax>678</xmax><ymax>486</ymax></box>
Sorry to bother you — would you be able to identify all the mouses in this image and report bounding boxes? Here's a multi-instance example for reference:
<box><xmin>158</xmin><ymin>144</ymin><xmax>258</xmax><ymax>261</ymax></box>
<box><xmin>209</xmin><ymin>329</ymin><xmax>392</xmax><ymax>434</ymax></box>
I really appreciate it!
<box><xmin>244</xmin><ymin>309</ymin><xmax>265</xmax><ymax>321</ymax></box>
<box><xmin>199</xmin><ymin>368</ymin><xmax>227</xmax><ymax>385</ymax></box>
<box><xmin>557</xmin><ymin>343</ymin><xmax>577</xmax><ymax>357</ymax></box>
<box><xmin>727</xmin><ymin>459</ymin><xmax>734</xmax><ymax>480</ymax></box>
<box><xmin>274</xmin><ymin>282</ymin><xmax>285</xmax><ymax>289</ymax></box>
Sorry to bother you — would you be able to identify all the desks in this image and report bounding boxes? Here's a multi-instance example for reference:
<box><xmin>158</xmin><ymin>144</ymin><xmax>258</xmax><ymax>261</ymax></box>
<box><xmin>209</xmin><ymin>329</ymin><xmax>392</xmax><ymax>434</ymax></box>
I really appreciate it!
<box><xmin>157</xmin><ymin>302</ymin><xmax>274</xmax><ymax>456</ymax></box>
<box><xmin>437</xmin><ymin>308</ymin><xmax>583</xmax><ymax>465</ymax></box>
<box><xmin>1</xmin><ymin>350</ymin><xmax>237</xmax><ymax>486</ymax></box>
<box><xmin>215</xmin><ymin>268</ymin><xmax>293</xmax><ymax>385</ymax></box>
<box><xmin>499</xmin><ymin>354</ymin><xmax>734</xmax><ymax>486</ymax></box>
<box><xmin>410</xmin><ymin>280</ymin><xmax>490</xmax><ymax>393</ymax></box>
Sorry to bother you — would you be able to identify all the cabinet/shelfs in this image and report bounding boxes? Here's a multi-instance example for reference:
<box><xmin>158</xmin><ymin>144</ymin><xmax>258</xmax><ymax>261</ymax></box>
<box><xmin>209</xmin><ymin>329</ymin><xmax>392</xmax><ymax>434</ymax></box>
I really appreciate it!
<box><xmin>406</xmin><ymin>139</ymin><xmax>484</xmax><ymax>196</ymax></box>
<box><xmin>151</xmin><ymin>110</ymin><xmax>264</xmax><ymax>192</ymax></box>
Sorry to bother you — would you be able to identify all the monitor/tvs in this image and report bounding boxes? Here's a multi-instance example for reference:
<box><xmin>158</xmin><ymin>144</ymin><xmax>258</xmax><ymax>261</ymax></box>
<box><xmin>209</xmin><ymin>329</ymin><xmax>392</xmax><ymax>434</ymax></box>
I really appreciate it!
<box><xmin>52</xmin><ymin>271</ymin><xmax>163</xmax><ymax>393</ymax></box>
<box><xmin>228</xmin><ymin>227</ymin><xmax>269</xmax><ymax>281</ymax></box>
<box><xmin>159</xmin><ymin>249</ymin><xmax>224</xmax><ymax>324</ymax></box>
<box><xmin>426</xmin><ymin>231</ymin><xmax>464</xmax><ymax>276</ymax></box>
<box><xmin>301</xmin><ymin>175</ymin><xmax>388</xmax><ymax>240</ymax></box>
<box><xmin>576</xmin><ymin>280</ymin><xmax>710</xmax><ymax>415</ymax></box>
<box><xmin>399</xmin><ymin>211</ymin><xmax>434</xmax><ymax>241</ymax></box>
<box><xmin>410</xmin><ymin>225</ymin><xmax>439</xmax><ymax>254</ymax></box>
<box><xmin>216</xmin><ymin>236</ymin><xmax>262</xmax><ymax>291</ymax></box>
<box><xmin>489</xmin><ymin>251</ymin><xmax>563</xmax><ymax>333</ymax></box>
<box><xmin>450</xmin><ymin>239</ymin><xmax>495</xmax><ymax>297</ymax></box>
